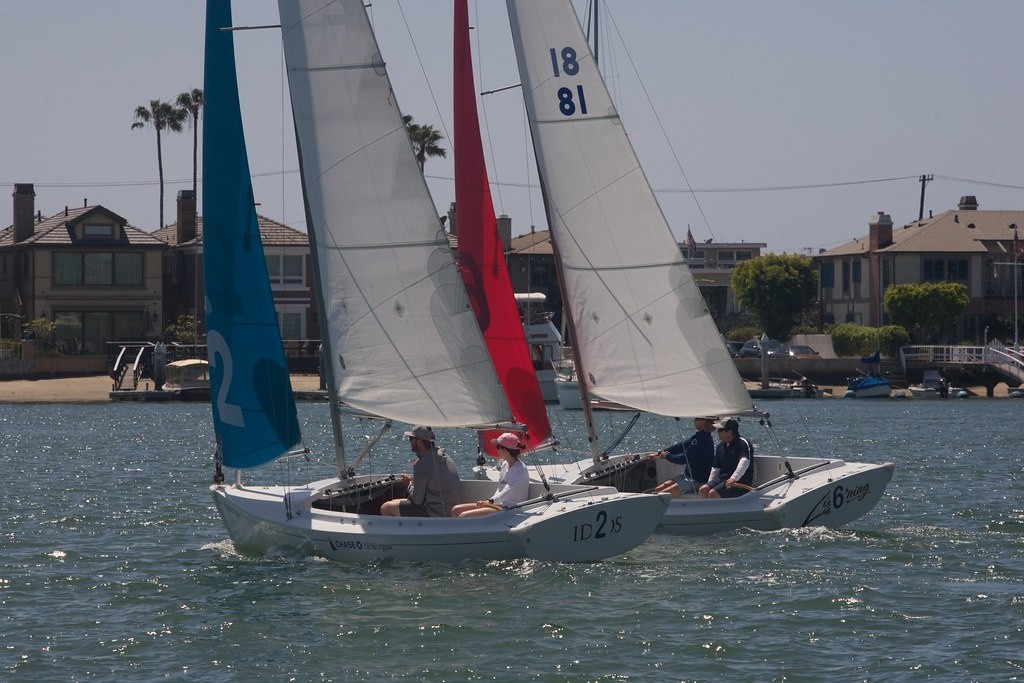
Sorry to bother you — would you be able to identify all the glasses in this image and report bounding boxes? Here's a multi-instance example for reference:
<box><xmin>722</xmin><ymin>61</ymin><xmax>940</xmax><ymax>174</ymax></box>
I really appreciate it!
<box><xmin>717</xmin><ymin>428</ymin><xmax>732</xmax><ymax>432</ymax></box>
<box><xmin>497</xmin><ymin>445</ymin><xmax>505</xmax><ymax>449</ymax></box>
<box><xmin>409</xmin><ymin>436</ymin><xmax>416</xmax><ymax>441</ymax></box>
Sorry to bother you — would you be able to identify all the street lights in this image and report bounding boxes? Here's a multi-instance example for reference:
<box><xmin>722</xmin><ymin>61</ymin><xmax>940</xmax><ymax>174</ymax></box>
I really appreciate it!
<box><xmin>1008</xmin><ymin>223</ymin><xmax>1018</xmax><ymax>365</ymax></box>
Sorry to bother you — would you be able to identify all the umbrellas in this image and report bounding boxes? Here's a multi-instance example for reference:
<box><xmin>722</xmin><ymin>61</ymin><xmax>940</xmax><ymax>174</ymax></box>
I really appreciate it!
<box><xmin>140</xmin><ymin>305</ymin><xmax>154</xmax><ymax>333</ymax></box>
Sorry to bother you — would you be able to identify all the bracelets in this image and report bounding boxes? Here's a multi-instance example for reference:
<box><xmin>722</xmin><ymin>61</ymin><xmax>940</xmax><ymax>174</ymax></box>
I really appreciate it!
<box><xmin>487</xmin><ymin>498</ymin><xmax>494</xmax><ymax>504</ymax></box>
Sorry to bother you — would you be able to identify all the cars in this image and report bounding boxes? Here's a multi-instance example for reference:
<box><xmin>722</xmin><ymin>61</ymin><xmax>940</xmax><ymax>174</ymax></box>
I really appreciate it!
<box><xmin>727</xmin><ymin>340</ymin><xmax>823</xmax><ymax>359</ymax></box>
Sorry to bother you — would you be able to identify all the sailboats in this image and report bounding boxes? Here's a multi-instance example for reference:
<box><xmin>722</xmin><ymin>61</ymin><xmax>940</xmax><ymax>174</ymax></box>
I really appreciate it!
<box><xmin>453</xmin><ymin>0</ymin><xmax>895</xmax><ymax>534</ymax></box>
<box><xmin>201</xmin><ymin>0</ymin><xmax>673</xmax><ymax>565</ymax></box>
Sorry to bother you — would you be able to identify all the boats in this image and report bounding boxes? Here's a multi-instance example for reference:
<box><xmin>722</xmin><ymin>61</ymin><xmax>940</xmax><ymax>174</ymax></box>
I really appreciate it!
<box><xmin>844</xmin><ymin>300</ymin><xmax>892</xmax><ymax>399</ymax></box>
<box><xmin>742</xmin><ymin>370</ymin><xmax>825</xmax><ymax>398</ymax></box>
<box><xmin>107</xmin><ymin>341</ymin><xmax>212</xmax><ymax>402</ymax></box>
<box><xmin>908</xmin><ymin>384</ymin><xmax>963</xmax><ymax>400</ymax></box>
<box><xmin>514</xmin><ymin>293</ymin><xmax>608</xmax><ymax>409</ymax></box>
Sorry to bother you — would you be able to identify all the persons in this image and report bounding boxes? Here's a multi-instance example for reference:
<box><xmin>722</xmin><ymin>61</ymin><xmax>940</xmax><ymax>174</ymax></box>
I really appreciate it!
<box><xmin>646</xmin><ymin>416</ymin><xmax>719</xmax><ymax>497</ymax></box>
<box><xmin>380</xmin><ymin>425</ymin><xmax>460</xmax><ymax>517</ymax></box>
<box><xmin>451</xmin><ymin>433</ymin><xmax>529</xmax><ymax>517</ymax></box>
<box><xmin>699</xmin><ymin>417</ymin><xmax>754</xmax><ymax>498</ymax></box>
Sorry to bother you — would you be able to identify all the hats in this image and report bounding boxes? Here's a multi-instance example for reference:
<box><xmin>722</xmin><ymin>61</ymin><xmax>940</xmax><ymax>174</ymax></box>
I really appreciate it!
<box><xmin>712</xmin><ymin>417</ymin><xmax>738</xmax><ymax>430</ymax></box>
<box><xmin>404</xmin><ymin>425</ymin><xmax>435</xmax><ymax>442</ymax></box>
<box><xmin>490</xmin><ymin>433</ymin><xmax>520</xmax><ymax>450</ymax></box>
